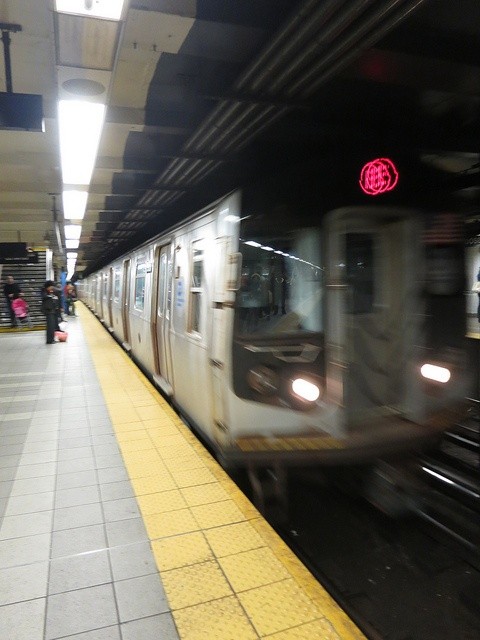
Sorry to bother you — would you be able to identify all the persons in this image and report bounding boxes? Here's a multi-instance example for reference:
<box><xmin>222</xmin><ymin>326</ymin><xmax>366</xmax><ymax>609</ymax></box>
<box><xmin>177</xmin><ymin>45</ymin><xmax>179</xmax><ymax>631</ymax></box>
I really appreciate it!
<box><xmin>41</xmin><ymin>280</ymin><xmax>58</xmax><ymax>344</ymax></box>
<box><xmin>4</xmin><ymin>275</ymin><xmax>25</xmax><ymax>326</ymax></box>
<box><xmin>54</xmin><ymin>281</ymin><xmax>78</xmax><ymax>322</ymax></box>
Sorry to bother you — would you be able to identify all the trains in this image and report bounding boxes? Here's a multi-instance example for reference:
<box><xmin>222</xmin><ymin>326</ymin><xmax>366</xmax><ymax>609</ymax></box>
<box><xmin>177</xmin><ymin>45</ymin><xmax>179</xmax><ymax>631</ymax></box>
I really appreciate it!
<box><xmin>75</xmin><ymin>157</ymin><xmax>471</xmax><ymax>518</ymax></box>
